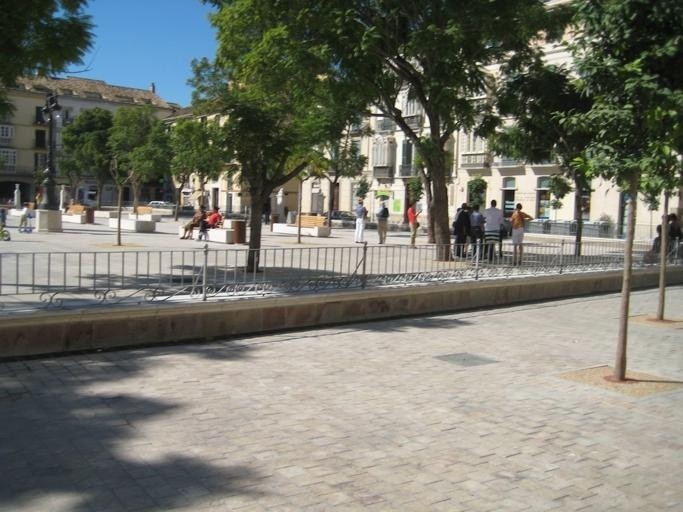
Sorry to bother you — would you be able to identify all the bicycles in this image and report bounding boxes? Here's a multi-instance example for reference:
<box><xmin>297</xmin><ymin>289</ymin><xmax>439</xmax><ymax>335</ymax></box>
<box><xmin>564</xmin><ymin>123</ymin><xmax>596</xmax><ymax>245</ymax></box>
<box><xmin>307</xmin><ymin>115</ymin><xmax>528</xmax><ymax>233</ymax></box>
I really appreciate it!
<box><xmin>0</xmin><ymin>222</ymin><xmax>10</xmax><ymax>241</ymax></box>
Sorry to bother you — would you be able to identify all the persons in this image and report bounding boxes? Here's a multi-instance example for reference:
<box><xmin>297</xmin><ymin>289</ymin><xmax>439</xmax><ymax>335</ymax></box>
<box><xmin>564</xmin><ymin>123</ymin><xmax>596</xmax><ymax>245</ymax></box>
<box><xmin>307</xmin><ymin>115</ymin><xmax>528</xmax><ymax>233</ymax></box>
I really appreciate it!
<box><xmin>407</xmin><ymin>201</ymin><xmax>422</xmax><ymax>249</ymax></box>
<box><xmin>653</xmin><ymin>214</ymin><xmax>683</xmax><ymax>254</ymax></box>
<box><xmin>180</xmin><ymin>205</ymin><xmax>221</xmax><ymax>241</ymax></box>
<box><xmin>375</xmin><ymin>202</ymin><xmax>388</xmax><ymax>244</ymax></box>
<box><xmin>509</xmin><ymin>204</ymin><xmax>534</xmax><ymax>266</ymax></box>
<box><xmin>0</xmin><ymin>209</ymin><xmax>6</xmax><ymax>232</ymax></box>
<box><xmin>354</xmin><ymin>198</ymin><xmax>367</xmax><ymax>243</ymax></box>
<box><xmin>18</xmin><ymin>204</ymin><xmax>29</xmax><ymax>233</ymax></box>
<box><xmin>485</xmin><ymin>199</ymin><xmax>503</xmax><ymax>258</ymax></box>
<box><xmin>453</xmin><ymin>203</ymin><xmax>484</xmax><ymax>259</ymax></box>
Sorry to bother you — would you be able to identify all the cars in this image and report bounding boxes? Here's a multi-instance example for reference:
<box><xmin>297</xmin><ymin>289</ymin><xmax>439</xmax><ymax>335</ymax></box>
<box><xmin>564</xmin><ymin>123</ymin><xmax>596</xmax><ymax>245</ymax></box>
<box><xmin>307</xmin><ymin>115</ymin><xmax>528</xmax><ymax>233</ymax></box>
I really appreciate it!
<box><xmin>173</xmin><ymin>205</ymin><xmax>194</xmax><ymax>215</ymax></box>
<box><xmin>147</xmin><ymin>200</ymin><xmax>167</xmax><ymax>207</ymax></box>
<box><xmin>321</xmin><ymin>210</ymin><xmax>370</xmax><ymax>229</ymax></box>
<box><xmin>296</xmin><ymin>212</ymin><xmax>320</xmax><ymax>216</ymax></box>
<box><xmin>269</xmin><ymin>213</ymin><xmax>280</xmax><ymax>223</ymax></box>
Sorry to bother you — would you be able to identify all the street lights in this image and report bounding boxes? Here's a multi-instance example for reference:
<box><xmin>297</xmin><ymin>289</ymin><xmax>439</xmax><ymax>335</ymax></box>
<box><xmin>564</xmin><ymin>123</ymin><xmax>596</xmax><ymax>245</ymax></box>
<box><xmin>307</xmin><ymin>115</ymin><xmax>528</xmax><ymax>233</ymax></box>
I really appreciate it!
<box><xmin>40</xmin><ymin>90</ymin><xmax>62</xmax><ymax>210</ymax></box>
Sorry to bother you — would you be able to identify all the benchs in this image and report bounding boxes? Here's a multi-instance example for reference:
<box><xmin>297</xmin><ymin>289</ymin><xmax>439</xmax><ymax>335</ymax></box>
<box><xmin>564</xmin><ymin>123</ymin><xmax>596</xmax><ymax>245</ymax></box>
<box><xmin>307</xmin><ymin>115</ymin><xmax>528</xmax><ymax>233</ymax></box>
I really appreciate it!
<box><xmin>286</xmin><ymin>214</ymin><xmax>330</xmax><ymax>235</ymax></box>
<box><xmin>60</xmin><ymin>206</ymin><xmax>161</xmax><ymax>232</ymax></box>
<box><xmin>180</xmin><ymin>210</ymin><xmax>225</xmax><ymax>242</ymax></box>
